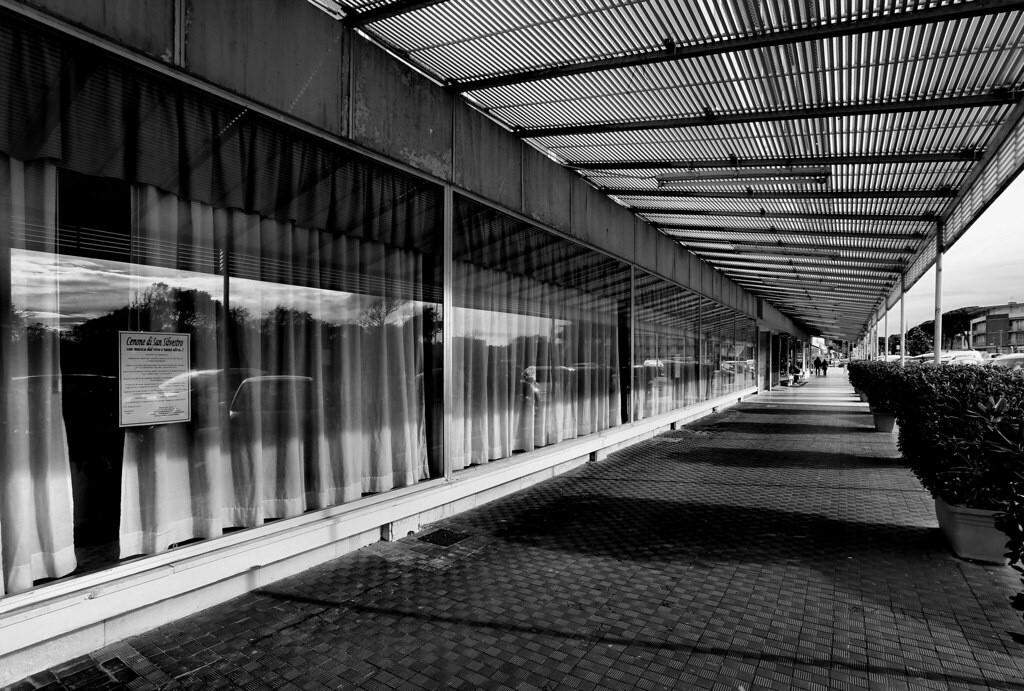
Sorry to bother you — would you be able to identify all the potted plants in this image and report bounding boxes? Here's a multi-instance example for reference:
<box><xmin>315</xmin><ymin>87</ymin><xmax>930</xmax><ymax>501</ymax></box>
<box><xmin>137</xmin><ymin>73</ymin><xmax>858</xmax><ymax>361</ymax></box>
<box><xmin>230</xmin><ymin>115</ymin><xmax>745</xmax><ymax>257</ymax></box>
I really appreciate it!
<box><xmin>846</xmin><ymin>359</ymin><xmax>899</xmax><ymax>431</ymax></box>
<box><xmin>894</xmin><ymin>361</ymin><xmax>1024</xmax><ymax>563</ymax></box>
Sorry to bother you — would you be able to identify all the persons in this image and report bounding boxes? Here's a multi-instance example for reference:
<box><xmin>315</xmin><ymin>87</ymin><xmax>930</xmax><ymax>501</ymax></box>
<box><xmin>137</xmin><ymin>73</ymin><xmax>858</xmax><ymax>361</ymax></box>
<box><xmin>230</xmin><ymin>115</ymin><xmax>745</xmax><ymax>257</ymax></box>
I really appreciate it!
<box><xmin>814</xmin><ymin>357</ymin><xmax>828</xmax><ymax>376</ymax></box>
